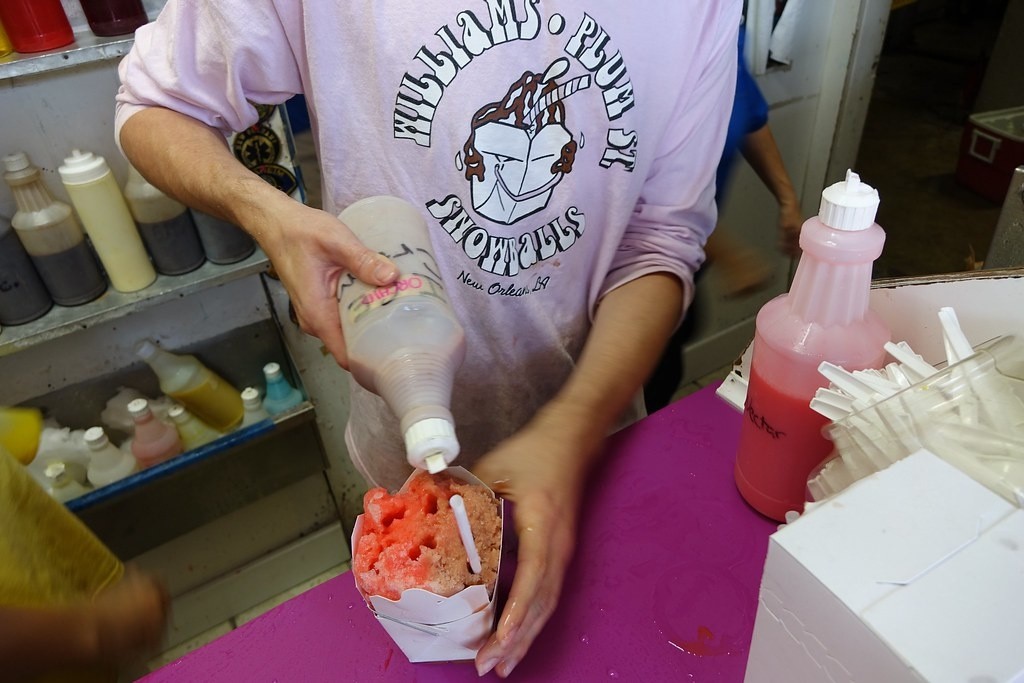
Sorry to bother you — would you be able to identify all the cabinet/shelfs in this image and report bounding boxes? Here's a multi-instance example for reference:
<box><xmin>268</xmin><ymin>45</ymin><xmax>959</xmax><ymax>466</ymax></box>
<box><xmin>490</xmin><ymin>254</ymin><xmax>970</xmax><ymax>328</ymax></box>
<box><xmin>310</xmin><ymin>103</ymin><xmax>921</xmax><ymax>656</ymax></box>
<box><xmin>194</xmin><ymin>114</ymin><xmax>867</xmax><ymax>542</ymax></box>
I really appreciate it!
<box><xmin>0</xmin><ymin>244</ymin><xmax>361</xmax><ymax>607</ymax></box>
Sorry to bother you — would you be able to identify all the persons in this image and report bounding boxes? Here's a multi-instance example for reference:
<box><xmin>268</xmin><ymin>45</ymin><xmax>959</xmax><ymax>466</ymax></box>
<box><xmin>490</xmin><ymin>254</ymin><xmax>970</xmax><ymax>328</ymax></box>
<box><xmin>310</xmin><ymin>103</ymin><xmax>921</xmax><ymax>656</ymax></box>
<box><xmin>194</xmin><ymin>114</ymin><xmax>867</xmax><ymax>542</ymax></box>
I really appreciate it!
<box><xmin>113</xmin><ymin>0</ymin><xmax>744</xmax><ymax>675</ymax></box>
<box><xmin>642</xmin><ymin>19</ymin><xmax>807</xmax><ymax>418</ymax></box>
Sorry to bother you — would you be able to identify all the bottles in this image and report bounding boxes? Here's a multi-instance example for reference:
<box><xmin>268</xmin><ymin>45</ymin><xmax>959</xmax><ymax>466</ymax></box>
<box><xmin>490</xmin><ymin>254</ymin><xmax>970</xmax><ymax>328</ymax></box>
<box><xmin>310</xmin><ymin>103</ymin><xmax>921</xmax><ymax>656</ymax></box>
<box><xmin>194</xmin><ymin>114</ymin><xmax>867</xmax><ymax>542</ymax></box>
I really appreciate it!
<box><xmin>735</xmin><ymin>166</ymin><xmax>893</xmax><ymax>522</ymax></box>
<box><xmin>12</xmin><ymin>340</ymin><xmax>300</xmax><ymax>505</ymax></box>
<box><xmin>190</xmin><ymin>202</ymin><xmax>258</xmax><ymax>265</ymax></box>
<box><xmin>0</xmin><ymin>0</ymin><xmax>75</xmax><ymax>57</ymax></box>
<box><xmin>57</xmin><ymin>149</ymin><xmax>157</xmax><ymax>293</ymax></box>
<box><xmin>0</xmin><ymin>211</ymin><xmax>54</xmax><ymax>326</ymax></box>
<box><xmin>79</xmin><ymin>0</ymin><xmax>149</xmax><ymax>37</ymax></box>
<box><xmin>331</xmin><ymin>193</ymin><xmax>467</xmax><ymax>475</ymax></box>
<box><xmin>1</xmin><ymin>150</ymin><xmax>106</xmax><ymax>306</ymax></box>
<box><xmin>123</xmin><ymin>154</ymin><xmax>205</xmax><ymax>276</ymax></box>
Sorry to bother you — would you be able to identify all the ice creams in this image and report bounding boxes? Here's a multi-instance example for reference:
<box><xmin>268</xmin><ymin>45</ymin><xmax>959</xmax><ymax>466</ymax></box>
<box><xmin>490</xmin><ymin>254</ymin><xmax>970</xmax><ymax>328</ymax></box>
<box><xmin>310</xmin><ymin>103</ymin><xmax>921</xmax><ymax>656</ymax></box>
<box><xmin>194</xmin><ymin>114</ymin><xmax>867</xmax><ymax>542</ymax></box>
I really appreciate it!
<box><xmin>352</xmin><ymin>470</ymin><xmax>501</xmax><ymax>602</ymax></box>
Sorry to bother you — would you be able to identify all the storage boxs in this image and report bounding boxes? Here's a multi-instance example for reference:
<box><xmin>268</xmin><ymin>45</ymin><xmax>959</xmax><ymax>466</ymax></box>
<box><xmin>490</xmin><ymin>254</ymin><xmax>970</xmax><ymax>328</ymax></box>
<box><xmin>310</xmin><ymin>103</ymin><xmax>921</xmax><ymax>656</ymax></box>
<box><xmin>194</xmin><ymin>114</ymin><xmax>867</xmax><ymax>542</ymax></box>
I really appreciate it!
<box><xmin>351</xmin><ymin>464</ymin><xmax>506</xmax><ymax>664</ymax></box>
<box><xmin>954</xmin><ymin>106</ymin><xmax>1024</xmax><ymax>207</ymax></box>
<box><xmin>739</xmin><ymin>447</ymin><xmax>1024</xmax><ymax>683</ymax></box>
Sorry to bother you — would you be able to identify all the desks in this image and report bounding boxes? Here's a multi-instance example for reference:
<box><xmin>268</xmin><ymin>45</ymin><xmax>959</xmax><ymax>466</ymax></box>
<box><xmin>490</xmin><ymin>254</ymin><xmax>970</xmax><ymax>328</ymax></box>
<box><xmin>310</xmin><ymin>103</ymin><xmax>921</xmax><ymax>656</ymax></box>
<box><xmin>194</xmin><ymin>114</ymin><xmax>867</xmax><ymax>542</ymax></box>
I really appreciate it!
<box><xmin>129</xmin><ymin>378</ymin><xmax>780</xmax><ymax>683</ymax></box>
